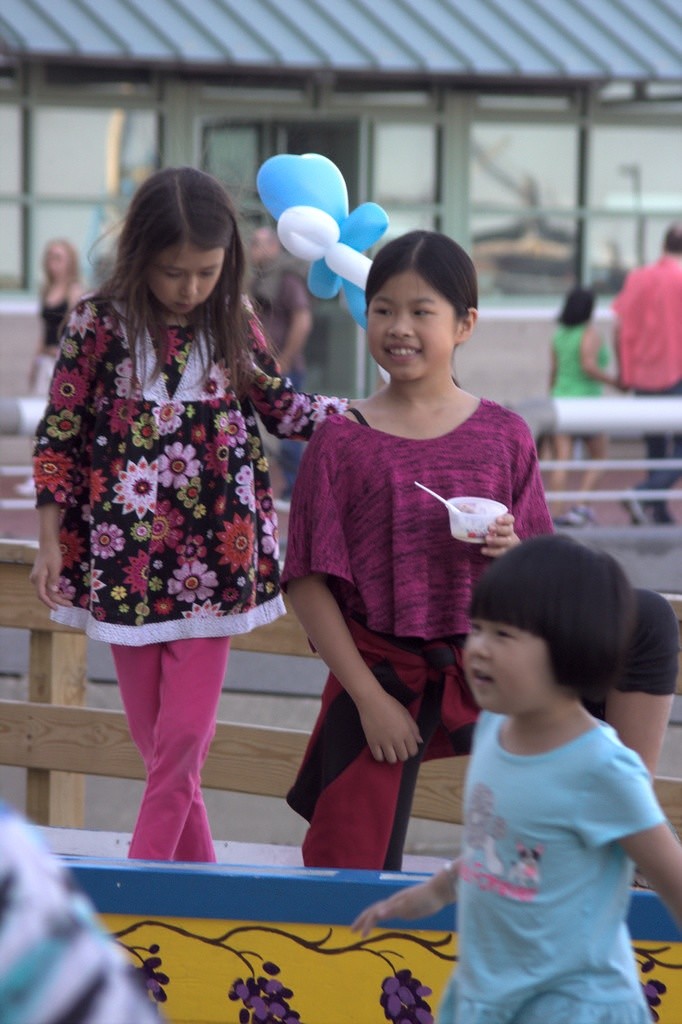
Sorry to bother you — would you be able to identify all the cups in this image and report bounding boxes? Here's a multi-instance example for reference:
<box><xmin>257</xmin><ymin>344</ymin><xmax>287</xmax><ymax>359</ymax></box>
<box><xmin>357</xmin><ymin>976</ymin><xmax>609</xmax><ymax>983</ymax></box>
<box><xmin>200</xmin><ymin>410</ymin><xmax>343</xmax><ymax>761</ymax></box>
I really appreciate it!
<box><xmin>445</xmin><ymin>496</ymin><xmax>508</xmax><ymax>544</ymax></box>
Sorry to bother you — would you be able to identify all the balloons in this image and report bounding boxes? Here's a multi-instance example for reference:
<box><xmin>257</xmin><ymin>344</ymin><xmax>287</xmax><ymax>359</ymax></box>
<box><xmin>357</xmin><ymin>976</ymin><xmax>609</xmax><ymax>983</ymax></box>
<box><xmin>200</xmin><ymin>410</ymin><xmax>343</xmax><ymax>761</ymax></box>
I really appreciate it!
<box><xmin>256</xmin><ymin>151</ymin><xmax>386</xmax><ymax>329</ymax></box>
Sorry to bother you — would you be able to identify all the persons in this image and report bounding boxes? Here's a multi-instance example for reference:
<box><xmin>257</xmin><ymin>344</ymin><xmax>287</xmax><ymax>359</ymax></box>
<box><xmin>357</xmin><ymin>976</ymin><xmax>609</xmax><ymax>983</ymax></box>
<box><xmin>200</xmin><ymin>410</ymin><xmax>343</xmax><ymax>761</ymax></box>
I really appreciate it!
<box><xmin>277</xmin><ymin>228</ymin><xmax>680</xmax><ymax>870</ymax></box>
<box><xmin>31</xmin><ymin>164</ymin><xmax>368</xmax><ymax>866</ymax></box>
<box><xmin>31</xmin><ymin>236</ymin><xmax>93</xmax><ymax>404</ymax></box>
<box><xmin>0</xmin><ymin>799</ymin><xmax>160</xmax><ymax>1023</ymax></box>
<box><xmin>612</xmin><ymin>222</ymin><xmax>682</xmax><ymax>524</ymax></box>
<box><xmin>352</xmin><ymin>533</ymin><xmax>681</xmax><ymax>1023</ymax></box>
<box><xmin>545</xmin><ymin>287</ymin><xmax>618</xmax><ymax>527</ymax></box>
<box><xmin>240</xmin><ymin>229</ymin><xmax>314</xmax><ymax>503</ymax></box>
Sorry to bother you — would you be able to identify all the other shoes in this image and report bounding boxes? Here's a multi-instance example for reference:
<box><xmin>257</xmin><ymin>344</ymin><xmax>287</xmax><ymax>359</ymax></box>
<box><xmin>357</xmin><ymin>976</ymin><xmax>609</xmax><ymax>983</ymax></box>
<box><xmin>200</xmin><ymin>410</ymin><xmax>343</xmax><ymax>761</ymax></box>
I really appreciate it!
<box><xmin>653</xmin><ymin>503</ymin><xmax>672</xmax><ymax>524</ymax></box>
<box><xmin>13</xmin><ymin>476</ymin><xmax>36</xmax><ymax>494</ymax></box>
<box><xmin>551</xmin><ymin>503</ymin><xmax>595</xmax><ymax>526</ymax></box>
<box><xmin>622</xmin><ymin>487</ymin><xmax>650</xmax><ymax>526</ymax></box>
